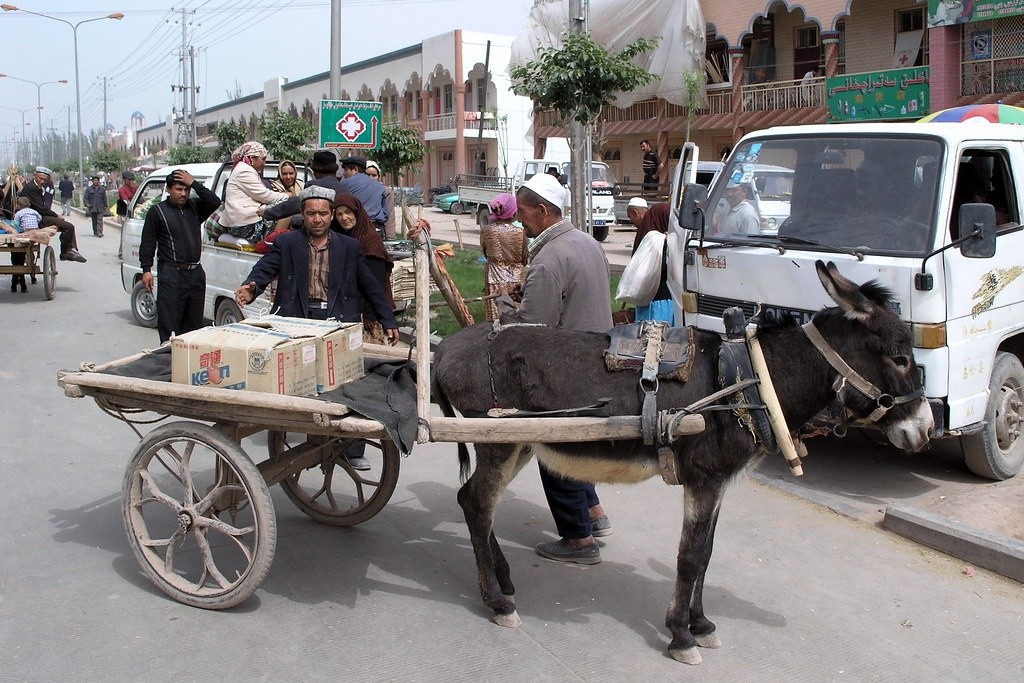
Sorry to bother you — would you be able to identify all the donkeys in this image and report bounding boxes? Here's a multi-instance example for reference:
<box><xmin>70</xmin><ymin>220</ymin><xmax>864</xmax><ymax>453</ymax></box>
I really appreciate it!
<box><xmin>427</xmin><ymin>260</ymin><xmax>935</xmax><ymax>664</ymax></box>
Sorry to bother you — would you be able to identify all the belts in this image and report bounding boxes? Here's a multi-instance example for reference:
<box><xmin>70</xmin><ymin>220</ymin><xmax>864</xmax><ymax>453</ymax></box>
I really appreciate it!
<box><xmin>159</xmin><ymin>257</ymin><xmax>201</xmax><ymax>271</ymax></box>
<box><xmin>308</xmin><ymin>301</ymin><xmax>328</xmax><ymax>309</ymax></box>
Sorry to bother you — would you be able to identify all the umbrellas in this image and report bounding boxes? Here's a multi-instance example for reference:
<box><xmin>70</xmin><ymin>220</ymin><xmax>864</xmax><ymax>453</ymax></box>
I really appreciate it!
<box><xmin>912</xmin><ymin>98</ymin><xmax>1024</xmax><ymax>128</ymax></box>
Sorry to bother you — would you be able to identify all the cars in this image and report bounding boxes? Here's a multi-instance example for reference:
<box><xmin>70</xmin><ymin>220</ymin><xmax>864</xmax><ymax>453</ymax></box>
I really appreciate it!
<box><xmin>429</xmin><ymin>188</ymin><xmax>482</xmax><ymax>206</ymax></box>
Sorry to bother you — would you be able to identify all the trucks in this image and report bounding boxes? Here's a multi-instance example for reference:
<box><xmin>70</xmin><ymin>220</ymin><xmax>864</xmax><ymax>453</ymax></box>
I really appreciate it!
<box><xmin>610</xmin><ymin>158</ymin><xmax>797</xmax><ymax>239</ymax></box>
<box><xmin>457</xmin><ymin>159</ymin><xmax>622</xmax><ymax>244</ymax></box>
<box><xmin>662</xmin><ymin>102</ymin><xmax>1023</xmax><ymax>482</ymax></box>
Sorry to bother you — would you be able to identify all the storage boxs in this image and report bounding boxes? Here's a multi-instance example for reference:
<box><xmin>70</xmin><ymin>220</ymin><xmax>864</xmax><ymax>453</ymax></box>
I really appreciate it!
<box><xmin>240</xmin><ymin>313</ymin><xmax>368</xmax><ymax>394</ymax></box>
<box><xmin>169</xmin><ymin>323</ymin><xmax>321</xmax><ymax>402</ymax></box>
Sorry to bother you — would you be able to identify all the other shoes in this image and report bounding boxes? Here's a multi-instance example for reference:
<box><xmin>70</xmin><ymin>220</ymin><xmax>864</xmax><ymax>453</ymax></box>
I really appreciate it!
<box><xmin>94</xmin><ymin>231</ymin><xmax>104</xmax><ymax>237</ymax></box>
<box><xmin>535</xmin><ymin>537</ymin><xmax>602</xmax><ymax>565</ymax></box>
<box><xmin>587</xmin><ymin>514</ymin><xmax>612</xmax><ymax>537</ymax></box>
<box><xmin>348</xmin><ymin>456</ymin><xmax>371</xmax><ymax>470</ymax></box>
<box><xmin>60</xmin><ymin>250</ymin><xmax>86</xmax><ymax>262</ymax></box>
<box><xmin>61</xmin><ymin>211</ymin><xmax>70</xmax><ymax>216</ymax></box>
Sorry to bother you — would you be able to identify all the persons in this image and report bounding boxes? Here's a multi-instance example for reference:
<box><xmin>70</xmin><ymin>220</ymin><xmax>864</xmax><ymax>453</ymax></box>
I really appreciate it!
<box><xmin>222</xmin><ymin>140</ymin><xmax>295</xmax><ymax>244</ymax></box>
<box><xmin>497</xmin><ymin>170</ymin><xmax>615</xmax><ymax>567</ymax></box>
<box><xmin>716</xmin><ymin>171</ymin><xmax>762</xmax><ymax>242</ymax></box>
<box><xmin>264</xmin><ymin>159</ymin><xmax>307</xmax><ymax>196</ymax></box>
<box><xmin>0</xmin><ymin>168</ymin><xmax>144</xmax><ymax>263</ymax></box>
<box><xmin>799</xmin><ymin>68</ymin><xmax>818</xmax><ymax>106</ymax></box>
<box><xmin>364</xmin><ymin>160</ymin><xmax>397</xmax><ymax>240</ymax></box>
<box><xmin>137</xmin><ymin>170</ymin><xmax>224</xmax><ymax>347</ymax></box>
<box><xmin>234</xmin><ymin>182</ymin><xmax>401</xmax><ymax>470</ymax></box>
<box><xmin>639</xmin><ymin>139</ymin><xmax>666</xmax><ymax>193</ymax></box>
<box><xmin>627</xmin><ymin>197</ymin><xmax>676</xmax><ymax>327</ymax></box>
<box><xmin>257</xmin><ymin>151</ymin><xmax>355</xmax><ymax>223</ymax></box>
<box><xmin>333</xmin><ymin>191</ymin><xmax>396</xmax><ymax>346</ymax></box>
<box><xmin>338</xmin><ymin>156</ymin><xmax>390</xmax><ymax>238</ymax></box>
<box><xmin>480</xmin><ymin>192</ymin><xmax>529</xmax><ymax>319</ymax></box>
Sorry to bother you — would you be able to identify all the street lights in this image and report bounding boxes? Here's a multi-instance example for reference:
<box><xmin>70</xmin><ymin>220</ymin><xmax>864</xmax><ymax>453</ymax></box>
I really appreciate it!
<box><xmin>1</xmin><ymin>3</ymin><xmax>124</xmax><ymax>212</ymax></box>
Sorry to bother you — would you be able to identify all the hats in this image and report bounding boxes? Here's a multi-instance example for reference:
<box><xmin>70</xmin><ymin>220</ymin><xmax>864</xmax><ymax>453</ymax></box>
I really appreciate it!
<box><xmin>35</xmin><ymin>166</ymin><xmax>52</xmax><ymax>176</ymax></box>
<box><xmin>339</xmin><ymin>156</ymin><xmax>366</xmax><ymax>168</ymax></box>
<box><xmin>628</xmin><ymin>196</ymin><xmax>648</xmax><ymax>208</ymax></box>
<box><xmin>122</xmin><ymin>171</ymin><xmax>134</xmax><ymax>180</ymax></box>
<box><xmin>312</xmin><ymin>151</ymin><xmax>336</xmax><ymax>166</ymax></box>
<box><xmin>522</xmin><ymin>172</ymin><xmax>566</xmax><ymax>210</ymax></box>
<box><xmin>725</xmin><ymin>169</ymin><xmax>743</xmax><ymax>189</ymax></box>
<box><xmin>298</xmin><ymin>185</ymin><xmax>337</xmax><ymax>204</ymax></box>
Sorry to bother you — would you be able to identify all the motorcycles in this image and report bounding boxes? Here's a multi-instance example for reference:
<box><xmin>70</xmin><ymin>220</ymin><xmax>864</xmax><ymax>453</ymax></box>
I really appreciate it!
<box><xmin>427</xmin><ymin>175</ymin><xmax>459</xmax><ymax>202</ymax></box>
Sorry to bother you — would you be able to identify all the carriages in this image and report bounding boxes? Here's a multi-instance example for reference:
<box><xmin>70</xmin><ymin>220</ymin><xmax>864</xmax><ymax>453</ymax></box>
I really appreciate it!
<box><xmin>0</xmin><ymin>170</ymin><xmax>59</xmax><ymax>300</ymax></box>
<box><xmin>56</xmin><ymin>192</ymin><xmax>941</xmax><ymax>671</ymax></box>
